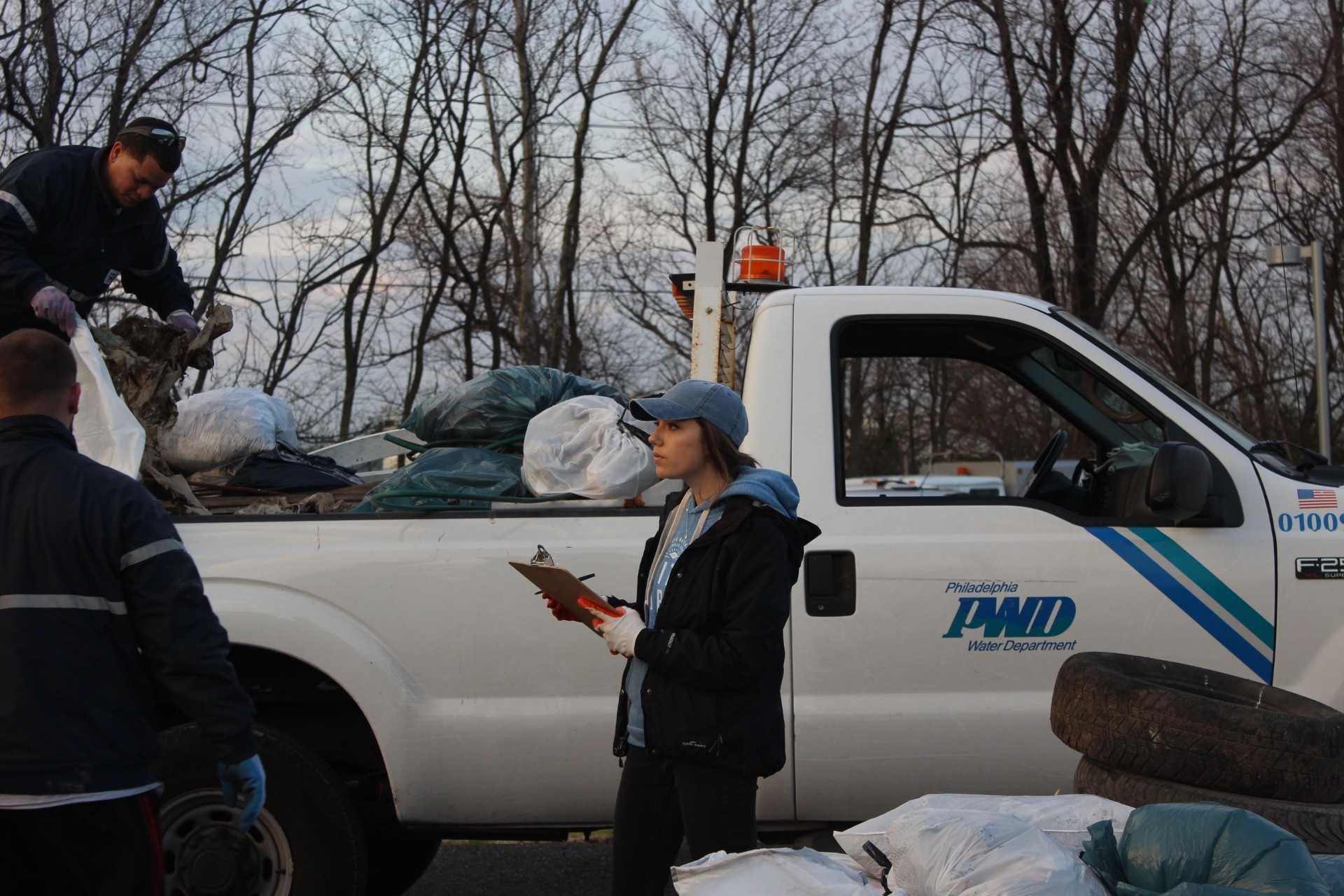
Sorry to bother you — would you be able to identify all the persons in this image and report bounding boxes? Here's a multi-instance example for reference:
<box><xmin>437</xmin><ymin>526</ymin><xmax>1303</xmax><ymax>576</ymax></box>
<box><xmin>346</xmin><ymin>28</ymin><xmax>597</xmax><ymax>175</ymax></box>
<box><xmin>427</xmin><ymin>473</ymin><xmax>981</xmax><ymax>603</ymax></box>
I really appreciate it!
<box><xmin>0</xmin><ymin>117</ymin><xmax>200</xmax><ymax>345</ymax></box>
<box><xmin>542</xmin><ymin>379</ymin><xmax>821</xmax><ymax>896</ymax></box>
<box><xmin>0</xmin><ymin>329</ymin><xmax>268</xmax><ymax>896</ymax></box>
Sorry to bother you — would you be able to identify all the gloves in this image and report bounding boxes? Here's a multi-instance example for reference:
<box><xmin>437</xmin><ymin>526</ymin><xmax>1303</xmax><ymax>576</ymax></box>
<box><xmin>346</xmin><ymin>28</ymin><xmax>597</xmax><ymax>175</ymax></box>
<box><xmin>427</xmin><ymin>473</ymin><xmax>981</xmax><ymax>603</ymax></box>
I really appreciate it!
<box><xmin>577</xmin><ymin>598</ymin><xmax>647</xmax><ymax>660</ymax></box>
<box><xmin>541</xmin><ymin>592</ymin><xmax>583</xmax><ymax>623</ymax></box>
<box><xmin>29</xmin><ymin>285</ymin><xmax>77</xmax><ymax>337</ymax></box>
<box><xmin>216</xmin><ymin>754</ymin><xmax>265</xmax><ymax>838</ymax></box>
<box><xmin>165</xmin><ymin>309</ymin><xmax>200</xmax><ymax>341</ymax></box>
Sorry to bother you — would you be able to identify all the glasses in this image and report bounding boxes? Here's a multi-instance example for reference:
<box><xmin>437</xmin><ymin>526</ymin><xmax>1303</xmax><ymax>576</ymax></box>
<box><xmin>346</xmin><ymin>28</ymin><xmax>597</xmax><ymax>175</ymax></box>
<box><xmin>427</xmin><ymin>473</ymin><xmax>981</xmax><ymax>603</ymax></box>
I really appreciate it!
<box><xmin>117</xmin><ymin>126</ymin><xmax>187</xmax><ymax>153</ymax></box>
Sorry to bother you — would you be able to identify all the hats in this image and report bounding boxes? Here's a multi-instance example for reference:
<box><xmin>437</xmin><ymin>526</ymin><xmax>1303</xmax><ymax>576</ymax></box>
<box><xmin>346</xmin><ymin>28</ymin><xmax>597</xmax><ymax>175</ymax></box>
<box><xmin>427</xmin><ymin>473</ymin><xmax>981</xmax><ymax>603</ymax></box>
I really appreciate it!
<box><xmin>627</xmin><ymin>379</ymin><xmax>750</xmax><ymax>448</ymax></box>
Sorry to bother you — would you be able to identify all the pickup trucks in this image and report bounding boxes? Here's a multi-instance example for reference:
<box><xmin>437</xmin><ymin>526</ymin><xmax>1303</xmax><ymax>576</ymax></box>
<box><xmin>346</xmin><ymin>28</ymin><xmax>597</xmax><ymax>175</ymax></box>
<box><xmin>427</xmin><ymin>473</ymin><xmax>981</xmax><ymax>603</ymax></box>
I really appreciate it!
<box><xmin>165</xmin><ymin>224</ymin><xmax>1344</xmax><ymax>896</ymax></box>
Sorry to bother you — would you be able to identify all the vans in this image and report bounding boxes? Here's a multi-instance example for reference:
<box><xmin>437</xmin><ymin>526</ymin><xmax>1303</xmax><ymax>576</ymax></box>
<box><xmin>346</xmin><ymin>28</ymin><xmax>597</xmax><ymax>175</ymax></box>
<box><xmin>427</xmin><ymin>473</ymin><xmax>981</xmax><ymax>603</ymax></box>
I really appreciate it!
<box><xmin>844</xmin><ymin>471</ymin><xmax>1005</xmax><ymax>498</ymax></box>
